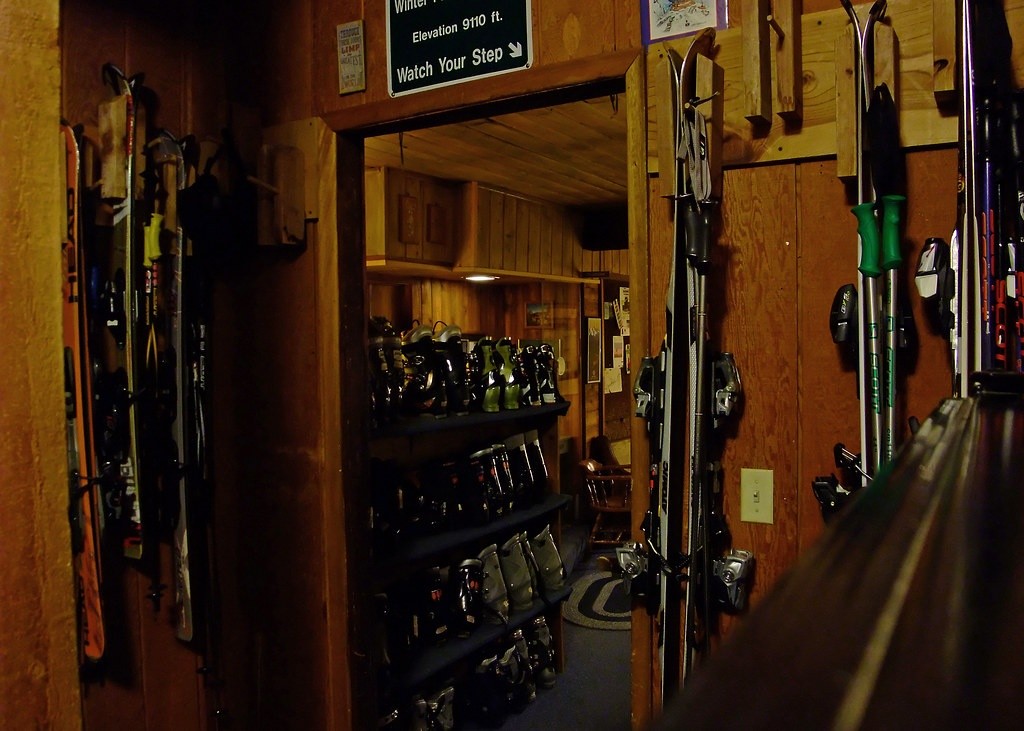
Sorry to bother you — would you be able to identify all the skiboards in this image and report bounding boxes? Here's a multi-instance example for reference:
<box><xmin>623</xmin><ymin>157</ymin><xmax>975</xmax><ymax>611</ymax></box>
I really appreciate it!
<box><xmin>616</xmin><ymin>1</ymin><xmax>1023</xmax><ymax>712</ymax></box>
<box><xmin>62</xmin><ymin>62</ymin><xmax>205</xmax><ymax>660</ymax></box>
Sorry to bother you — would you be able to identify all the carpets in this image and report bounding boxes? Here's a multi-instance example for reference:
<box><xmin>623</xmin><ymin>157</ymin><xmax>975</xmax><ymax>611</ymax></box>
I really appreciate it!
<box><xmin>562</xmin><ymin>570</ymin><xmax>631</xmax><ymax>630</ymax></box>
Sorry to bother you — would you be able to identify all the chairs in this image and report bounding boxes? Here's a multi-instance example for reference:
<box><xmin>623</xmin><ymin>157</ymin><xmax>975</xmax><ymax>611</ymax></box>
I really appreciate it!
<box><xmin>578</xmin><ymin>459</ymin><xmax>632</xmax><ymax>562</ymax></box>
<box><xmin>591</xmin><ymin>435</ymin><xmax>629</xmax><ymax>495</ymax></box>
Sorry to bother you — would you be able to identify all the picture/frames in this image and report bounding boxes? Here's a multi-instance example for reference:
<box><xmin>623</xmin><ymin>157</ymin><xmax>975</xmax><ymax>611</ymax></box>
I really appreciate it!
<box><xmin>640</xmin><ymin>0</ymin><xmax>728</xmax><ymax>46</ymax></box>
<box><xmin>583</xmin><ymin>317</ymin><xmax>601</xmax><ymax>382</ymax></box>
<box><xmin>523</xmin><ymin>300</ymin><xmax>554</xmax><ymax>330</ymax></box>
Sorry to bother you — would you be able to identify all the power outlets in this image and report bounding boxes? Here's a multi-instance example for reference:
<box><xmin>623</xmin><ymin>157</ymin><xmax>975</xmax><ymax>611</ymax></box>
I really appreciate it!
<box><xmin>741</xmin><ymin>469</ymin><xmax>773</xmax><ymax>524</ymax></box>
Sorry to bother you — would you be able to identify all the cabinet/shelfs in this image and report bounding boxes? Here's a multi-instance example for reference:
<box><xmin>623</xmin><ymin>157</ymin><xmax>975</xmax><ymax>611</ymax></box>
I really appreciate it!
<box><xmin>455</xmin><ymin>184</ymin><xmax>600</xmax><ymax>287</ymax></box>
<box><xmin>365</xmin><ymin>166</ymin><xmax>454</xmax><ymax>280</ymax></box>
<box><xmin>601</xmin><ymin>249</ymin><xmax>629</xmax><ymax>283</ymax></box>
<box><xmin>343</xmin><ymin>402</ymin><xmax>569</xmax><ymax>731</ymax></box>
<box><xmin>645</xmin><ymin>396</ymin><xmax>1022</xmax><ymax>731</ymax></box>
